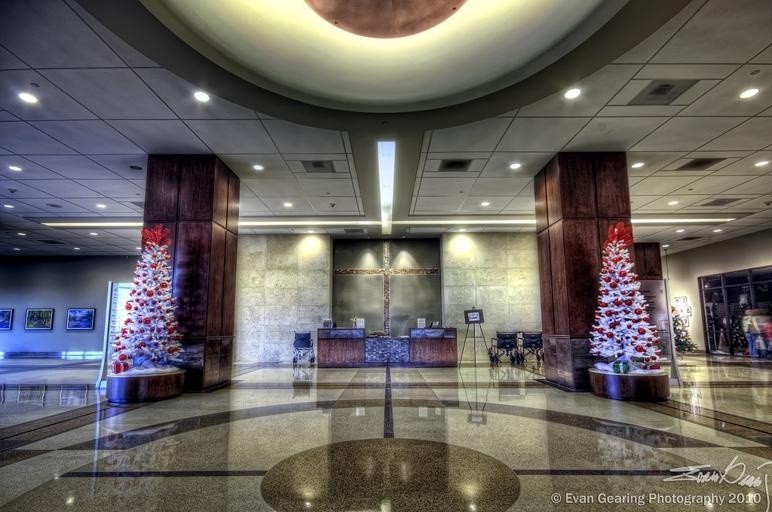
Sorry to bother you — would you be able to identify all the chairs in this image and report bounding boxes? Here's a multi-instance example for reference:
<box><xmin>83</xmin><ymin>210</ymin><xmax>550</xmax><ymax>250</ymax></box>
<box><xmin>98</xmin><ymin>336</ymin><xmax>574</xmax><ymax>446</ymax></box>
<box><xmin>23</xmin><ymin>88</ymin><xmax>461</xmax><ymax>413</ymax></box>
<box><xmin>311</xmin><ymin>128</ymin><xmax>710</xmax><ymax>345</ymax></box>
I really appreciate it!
<box><xmin>292</xmin><ymin>331</ymin><xmax>316</xmax><ymax>365</ymax></box>
<box><xmin>488</xmin><ymin>330</ymin><xmax>545</xmax><ymax>366</ymax></box>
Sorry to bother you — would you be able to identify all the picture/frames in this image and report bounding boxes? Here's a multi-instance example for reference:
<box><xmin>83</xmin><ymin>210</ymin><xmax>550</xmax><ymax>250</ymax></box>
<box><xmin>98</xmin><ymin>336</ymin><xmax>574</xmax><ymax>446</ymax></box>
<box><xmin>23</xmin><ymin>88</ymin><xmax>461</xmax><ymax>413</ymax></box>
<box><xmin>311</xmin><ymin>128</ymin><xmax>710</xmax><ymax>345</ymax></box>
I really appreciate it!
<box><xmin>58</xmin><ymin>383</ymin><xmax>89</xmax><ymax>408</ymax></box>
<box><xmin>24</xmin><ymin>307</ymin><xmax>55</xmax><ymax>331</ymax></box>
<box><xmin>0</xmin><ymin>308</ymin><xmax>14</xmax><ymax>331</ymax></box>
<box><xmin>16</xmin><ymin>384</ymin><xmax>48</xmax><ymax>408</ymax></box>
<box><xmin>672</xmin><ymin>296</ymin><xmax>693</xmax><ymax>327</ymax></box>
<box><xmin>65</xmin><ymin>307</ymin><xmax>96</xmax><ymax>331</ymax></box>
<box><xmin>0</xmin><ymin>383</ymin><xmax>5</xmax><ymax>405</ymax></box>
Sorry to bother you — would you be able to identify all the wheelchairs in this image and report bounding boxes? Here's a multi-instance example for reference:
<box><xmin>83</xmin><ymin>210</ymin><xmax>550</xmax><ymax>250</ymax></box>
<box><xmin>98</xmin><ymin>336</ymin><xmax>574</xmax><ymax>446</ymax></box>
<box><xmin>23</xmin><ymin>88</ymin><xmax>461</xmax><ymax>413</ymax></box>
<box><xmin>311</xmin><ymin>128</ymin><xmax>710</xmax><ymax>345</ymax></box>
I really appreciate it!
<box><xmin>491</xmin><ymin>328</ymin><xmax>545</xmax><ymax>366</ymax></box>
<box><xmin>493</xmin><ymin>366</ymin><xmax>546</xmax><ymax>402</ymax></box>
<box><xmin>292</xmin><ymin>368</ymin><xmax>314</xmax><ymax>399</ymax></box>
<box><xmin>291</xmin><ymin>330</ymin><xmax>316</xmax><ymax>368</ymax></box>
